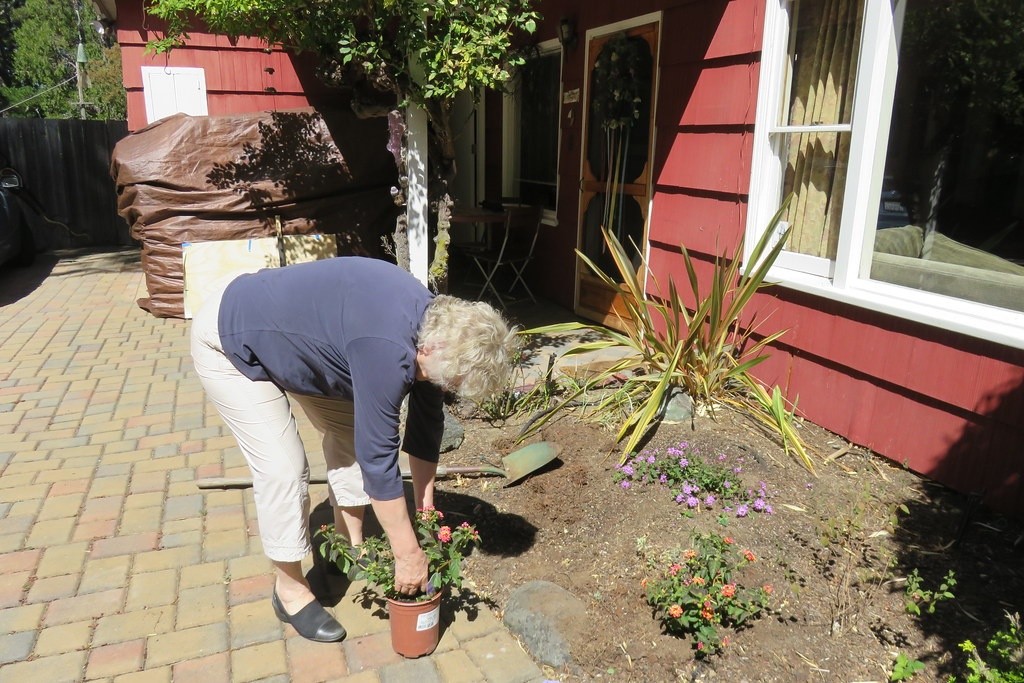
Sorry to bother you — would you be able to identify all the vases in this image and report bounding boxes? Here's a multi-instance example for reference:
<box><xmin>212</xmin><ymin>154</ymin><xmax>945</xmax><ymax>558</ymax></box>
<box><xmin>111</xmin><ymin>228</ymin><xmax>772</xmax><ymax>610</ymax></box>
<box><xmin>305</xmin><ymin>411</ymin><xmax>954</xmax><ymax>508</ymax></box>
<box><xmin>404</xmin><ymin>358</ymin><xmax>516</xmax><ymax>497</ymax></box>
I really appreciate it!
<box><xmin>387</xmin><ymin>590</ymin><xmax>441</xmax><ymax>658</ymax></box>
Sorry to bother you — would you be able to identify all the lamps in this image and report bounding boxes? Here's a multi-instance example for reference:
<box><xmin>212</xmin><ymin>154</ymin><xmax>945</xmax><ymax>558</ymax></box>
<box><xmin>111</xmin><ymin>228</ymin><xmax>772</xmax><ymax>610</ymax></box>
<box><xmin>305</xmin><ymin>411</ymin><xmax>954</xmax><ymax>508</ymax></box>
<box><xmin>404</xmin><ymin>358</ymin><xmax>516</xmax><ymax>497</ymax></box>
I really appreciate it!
<box><xmin>94</xmin><ymin>18</ymin><xmax>116</xmax><ymax>35</ymax></box>
<box><xmin>555</xmin><ymin>17</ymin><xmax>578</xmax><ymax>60</ymax></box>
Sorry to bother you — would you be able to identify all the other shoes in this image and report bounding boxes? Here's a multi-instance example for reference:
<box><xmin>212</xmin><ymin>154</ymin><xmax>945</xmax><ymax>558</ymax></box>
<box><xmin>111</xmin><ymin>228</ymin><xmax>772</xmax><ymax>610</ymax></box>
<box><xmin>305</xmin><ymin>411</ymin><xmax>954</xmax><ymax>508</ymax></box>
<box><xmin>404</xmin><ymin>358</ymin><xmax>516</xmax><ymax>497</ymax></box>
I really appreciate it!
<box><xmin>272</xmin><ymin>583</ymin><xmax>346</xmax><ymax>642</ymax></box>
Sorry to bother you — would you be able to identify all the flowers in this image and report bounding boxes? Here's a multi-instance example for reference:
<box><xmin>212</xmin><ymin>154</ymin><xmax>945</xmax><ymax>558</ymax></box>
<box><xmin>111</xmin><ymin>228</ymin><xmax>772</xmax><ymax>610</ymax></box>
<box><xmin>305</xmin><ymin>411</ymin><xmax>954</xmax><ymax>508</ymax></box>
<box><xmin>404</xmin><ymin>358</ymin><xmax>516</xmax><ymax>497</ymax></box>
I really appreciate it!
<box><xmin>310</xmin><ymin>506</ymin><xmax>483</xmax><ymax>602</ymax></box>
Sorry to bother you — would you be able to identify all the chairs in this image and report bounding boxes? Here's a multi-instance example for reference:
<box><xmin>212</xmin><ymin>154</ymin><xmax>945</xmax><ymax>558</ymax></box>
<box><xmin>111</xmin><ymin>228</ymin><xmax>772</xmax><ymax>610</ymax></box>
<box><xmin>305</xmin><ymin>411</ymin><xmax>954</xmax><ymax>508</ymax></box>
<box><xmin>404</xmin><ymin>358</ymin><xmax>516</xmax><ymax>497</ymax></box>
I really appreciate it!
<box><xmin>453</xmin><ymin>197</ymin><xmax>538</xmax><ymax>317</ymax></box>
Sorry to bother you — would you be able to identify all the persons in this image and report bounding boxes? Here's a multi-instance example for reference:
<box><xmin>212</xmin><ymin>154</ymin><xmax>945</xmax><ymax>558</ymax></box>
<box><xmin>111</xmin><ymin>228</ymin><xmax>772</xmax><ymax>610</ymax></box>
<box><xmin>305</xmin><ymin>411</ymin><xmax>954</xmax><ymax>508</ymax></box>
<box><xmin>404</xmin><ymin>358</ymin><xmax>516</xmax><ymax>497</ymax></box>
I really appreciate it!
<box><xmin>189</xmin><ymin>257</ymin><xmax>525</xmax><ymax>645</ymax></box>
